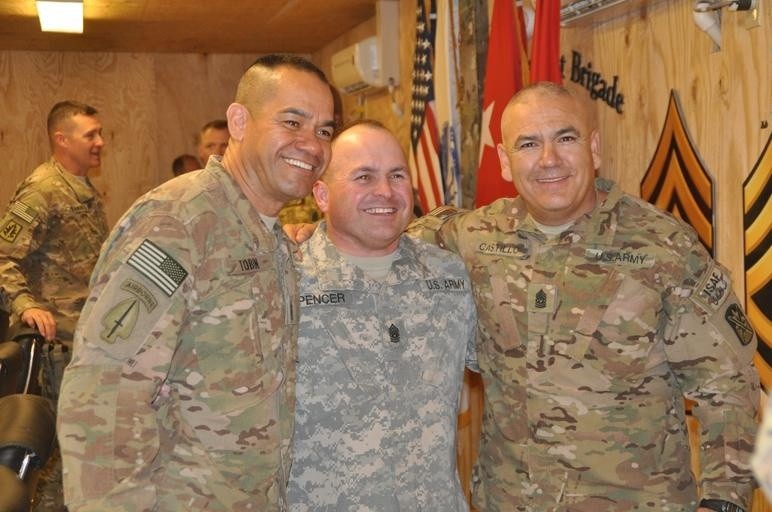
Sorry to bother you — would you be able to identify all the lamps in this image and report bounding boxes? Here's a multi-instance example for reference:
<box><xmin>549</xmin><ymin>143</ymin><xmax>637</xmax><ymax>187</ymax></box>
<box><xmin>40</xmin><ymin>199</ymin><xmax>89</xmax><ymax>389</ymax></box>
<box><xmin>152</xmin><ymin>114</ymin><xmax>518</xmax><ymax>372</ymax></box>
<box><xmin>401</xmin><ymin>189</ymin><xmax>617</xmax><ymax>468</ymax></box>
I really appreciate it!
<box><xmin>35</xmin><ymin>1</ymin><xmax>87</xmax><ymax>35</ymax></box>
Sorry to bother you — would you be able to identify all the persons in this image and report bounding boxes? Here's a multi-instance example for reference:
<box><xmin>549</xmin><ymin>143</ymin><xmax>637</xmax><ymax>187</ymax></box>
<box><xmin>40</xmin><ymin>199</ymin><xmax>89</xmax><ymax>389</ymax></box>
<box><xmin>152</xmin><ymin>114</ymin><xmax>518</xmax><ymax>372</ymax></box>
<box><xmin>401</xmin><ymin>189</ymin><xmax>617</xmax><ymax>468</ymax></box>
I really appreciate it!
<box><xmin>280</xmin><ymin>79</ymin><xmax>762</xmax><ymax>512</ymax></box>
<box><xmin>279</xmin><ymin>122</ymin><xmax>482</xmax><ymax>511</ymax></box>
<box><xmin>55</xmin><ymin>54</ymin><xmax>337</xmax><ymax>511</ymax></box>
<box><xmin>1</xmin><ymin>97</ymin><xmax>106</xmax><ymax>509</ymax></box>
<box><xmin>198</xmin><ymin>118</ymin><xmax>231</xmax><ymax>168</ymax></box>
<box><xmin>173</xmin><ymin>154</ymin><xmax>202</xmax><ymax>178</ymax></box>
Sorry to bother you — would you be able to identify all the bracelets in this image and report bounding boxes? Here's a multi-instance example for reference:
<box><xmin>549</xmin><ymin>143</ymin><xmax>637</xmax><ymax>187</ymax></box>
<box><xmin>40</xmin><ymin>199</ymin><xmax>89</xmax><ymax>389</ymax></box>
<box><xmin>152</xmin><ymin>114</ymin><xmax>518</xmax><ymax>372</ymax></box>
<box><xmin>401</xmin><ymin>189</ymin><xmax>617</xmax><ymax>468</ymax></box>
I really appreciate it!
<box><xmin>697</xmin><ymin>495</ymin><xmax>747</xmax><ymax>511</ymax></box>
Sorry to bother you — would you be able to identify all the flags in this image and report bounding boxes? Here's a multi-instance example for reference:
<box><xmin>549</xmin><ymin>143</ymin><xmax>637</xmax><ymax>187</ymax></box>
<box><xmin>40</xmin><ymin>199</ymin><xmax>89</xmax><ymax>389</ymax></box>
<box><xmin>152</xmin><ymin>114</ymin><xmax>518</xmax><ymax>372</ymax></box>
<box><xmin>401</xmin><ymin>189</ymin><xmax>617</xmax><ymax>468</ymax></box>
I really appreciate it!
<box><xmin>457</xmin><ymin>1</ymin><xmax>532</xmax><ymax>216</ymax></box>
<box><xmin>526</xmin><ymin>1</ymin><xmax>565</xmax><ymax>84</ymax></box>
<box><xmin>405</xmin><ymin>2</ymin><xmax>461</xmax><ymax>216</ymax></box>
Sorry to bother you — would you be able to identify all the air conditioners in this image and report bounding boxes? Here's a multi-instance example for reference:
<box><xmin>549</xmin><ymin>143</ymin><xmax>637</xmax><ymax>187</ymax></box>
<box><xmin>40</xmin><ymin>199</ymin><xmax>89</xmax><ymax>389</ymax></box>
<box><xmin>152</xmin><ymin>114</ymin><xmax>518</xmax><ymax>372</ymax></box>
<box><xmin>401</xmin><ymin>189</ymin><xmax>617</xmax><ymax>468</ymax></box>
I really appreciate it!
<box><xmin>529</xmin><ymin>0</ymin><xmax>665</xmax><ymax>29</ymax></box>
<box><xmin>329</xmin><ymin>34</ymin><xmax>390</xmax><ymax>98</ymax></box>
